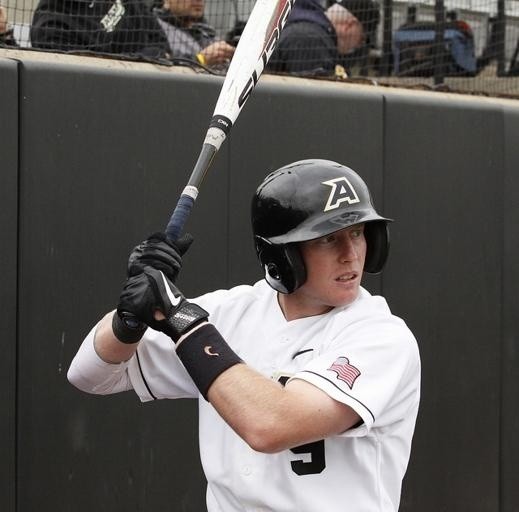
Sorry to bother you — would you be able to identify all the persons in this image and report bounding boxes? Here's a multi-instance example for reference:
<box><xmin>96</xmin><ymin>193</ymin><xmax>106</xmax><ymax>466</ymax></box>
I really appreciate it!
<box><xmin>1</xmin><ymin>4</ymin><xmax>519</xmax><ymax>78</ymax></box>
<box><xmin>66</xmin><ymin>158</ymin><xmax>422</xmax><ymax>512</ymax></box>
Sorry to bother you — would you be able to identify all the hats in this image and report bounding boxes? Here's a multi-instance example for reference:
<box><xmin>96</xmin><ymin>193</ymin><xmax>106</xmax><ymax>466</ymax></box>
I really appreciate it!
<box><xmin>337</xmin><ymin>1</ymin><xmax>381</xmax><ymax>51</ymax></box>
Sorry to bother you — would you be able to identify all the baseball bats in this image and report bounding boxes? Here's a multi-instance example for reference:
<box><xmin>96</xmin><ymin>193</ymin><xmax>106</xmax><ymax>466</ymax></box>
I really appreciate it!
<box><xmin>121</xmin><ymin>1</ymin><xmax>298</xmax><ymax>324</ymax></box>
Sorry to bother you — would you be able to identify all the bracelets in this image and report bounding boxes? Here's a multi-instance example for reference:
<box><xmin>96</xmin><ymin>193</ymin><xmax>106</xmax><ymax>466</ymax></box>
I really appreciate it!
<box><xmin>175</xmin><ymin>322</ymin><xmax>243</xmax><ymax>401</ymax></box>
<box><xmin>112</xmin><ymin>312</ymin><xmax>147</xmax><ymax>344</ymax></box>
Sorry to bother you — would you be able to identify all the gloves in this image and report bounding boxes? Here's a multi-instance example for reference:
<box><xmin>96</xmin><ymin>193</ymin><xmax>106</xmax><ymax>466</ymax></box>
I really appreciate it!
<box><xmin>127</xmin><ymin>233</ymin><xmax>194</xmax><ymax>283</ymax></box>
<box><xmin>118</xmin><ymin>269</ymin><xmax>208</xmax><ymax>342</ymax></box>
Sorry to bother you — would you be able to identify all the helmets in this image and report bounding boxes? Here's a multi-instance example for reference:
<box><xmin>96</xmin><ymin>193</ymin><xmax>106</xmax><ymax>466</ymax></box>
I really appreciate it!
<box><xmin>253</xmin><ymin>158</ymin><xmax>394</xmax><ymax>294</ymax></box>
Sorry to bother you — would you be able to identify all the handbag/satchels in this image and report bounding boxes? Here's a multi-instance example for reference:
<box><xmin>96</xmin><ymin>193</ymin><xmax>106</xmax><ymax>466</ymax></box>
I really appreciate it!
<box><xmin>392</xmin><ymin>21</ymin><xmax>475</xmax><ymax>76</ymax></box>
